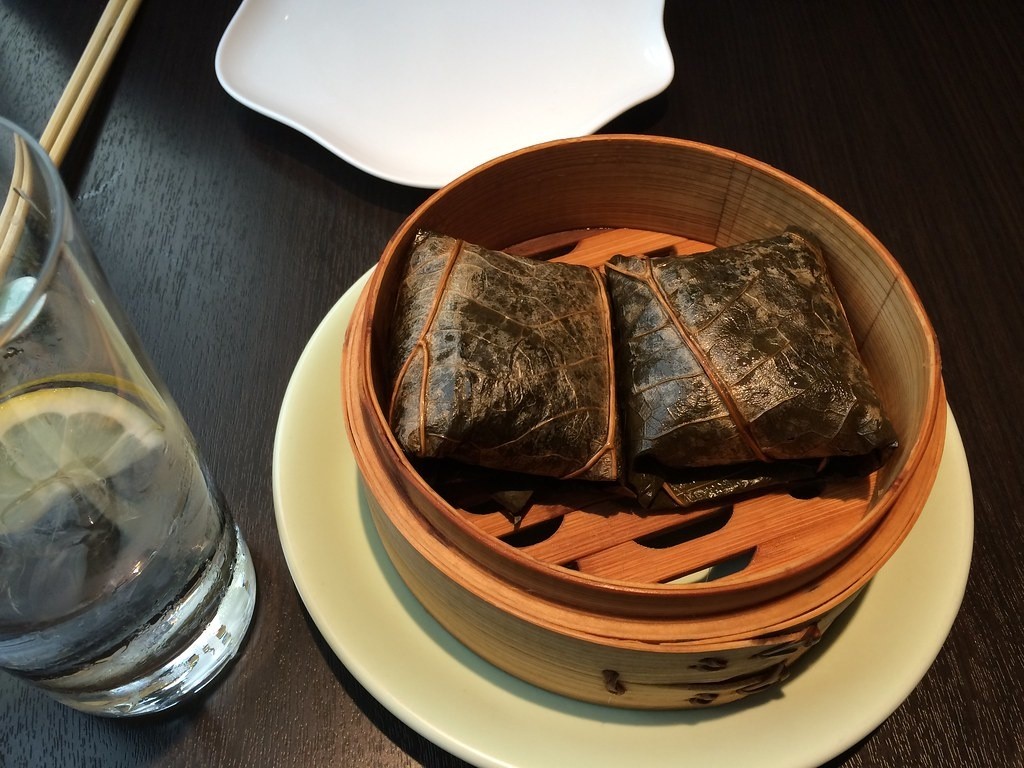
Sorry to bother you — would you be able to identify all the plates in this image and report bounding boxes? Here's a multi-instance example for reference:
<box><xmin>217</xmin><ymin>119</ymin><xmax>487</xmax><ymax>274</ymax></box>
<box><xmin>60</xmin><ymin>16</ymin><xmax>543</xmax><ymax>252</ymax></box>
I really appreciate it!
<box><xmin>215</xmin><ymin>0</ymin><xmax>675</xmax><ymax>188</ymax></box>
<box><xmin>270</xmin><ymin>262</ymin><xmax>977</xmax><ymax>768</ymax></box>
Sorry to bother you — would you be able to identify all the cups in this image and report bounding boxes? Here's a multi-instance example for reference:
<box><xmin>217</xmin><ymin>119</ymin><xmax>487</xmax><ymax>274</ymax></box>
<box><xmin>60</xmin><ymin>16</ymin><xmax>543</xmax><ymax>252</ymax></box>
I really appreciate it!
<box><xmin>0</xmin><ymin>114</ymin><xmax>256</xmax><ymax>717</ymax></box>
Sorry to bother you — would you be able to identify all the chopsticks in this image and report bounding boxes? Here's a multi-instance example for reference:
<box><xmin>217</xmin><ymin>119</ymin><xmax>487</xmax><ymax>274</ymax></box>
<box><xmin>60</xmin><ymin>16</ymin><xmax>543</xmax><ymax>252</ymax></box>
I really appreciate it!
<box><xmin>0</xmin><ymin>0</ymin><xmax>137</xmax><ymax>281</ymax></box>
